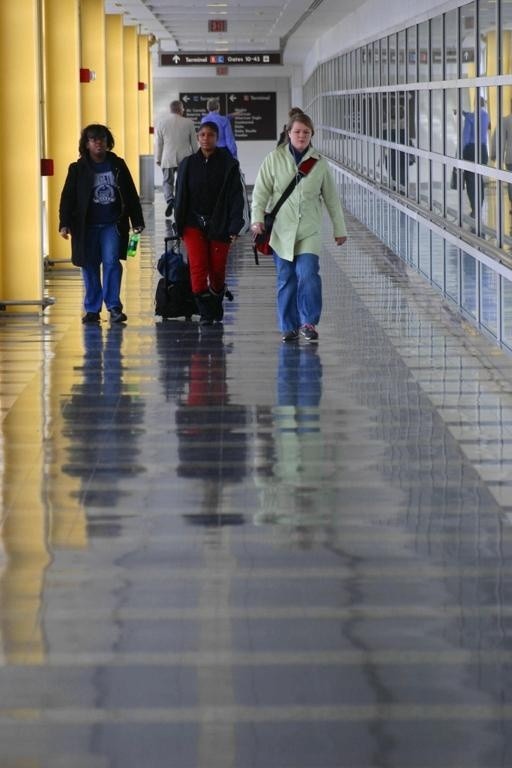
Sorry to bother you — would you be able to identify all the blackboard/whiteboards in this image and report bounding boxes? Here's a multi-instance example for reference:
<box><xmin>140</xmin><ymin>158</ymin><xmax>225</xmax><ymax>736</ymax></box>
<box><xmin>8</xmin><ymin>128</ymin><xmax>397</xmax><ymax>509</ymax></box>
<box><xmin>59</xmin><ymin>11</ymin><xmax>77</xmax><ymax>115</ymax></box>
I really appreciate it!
<box><xmin>179</xmin><ymin>92</ymin><xmax>277</xmax><ymax>140</ymax></box>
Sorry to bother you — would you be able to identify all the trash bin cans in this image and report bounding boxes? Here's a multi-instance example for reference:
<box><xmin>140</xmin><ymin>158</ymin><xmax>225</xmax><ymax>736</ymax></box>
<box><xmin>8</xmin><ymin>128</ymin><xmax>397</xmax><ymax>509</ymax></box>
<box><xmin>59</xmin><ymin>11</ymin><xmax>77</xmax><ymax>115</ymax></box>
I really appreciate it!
<box><xmin>139</xmin><ymin>155</ymin><xmax>155</xmax><ymax>204</ymax></box>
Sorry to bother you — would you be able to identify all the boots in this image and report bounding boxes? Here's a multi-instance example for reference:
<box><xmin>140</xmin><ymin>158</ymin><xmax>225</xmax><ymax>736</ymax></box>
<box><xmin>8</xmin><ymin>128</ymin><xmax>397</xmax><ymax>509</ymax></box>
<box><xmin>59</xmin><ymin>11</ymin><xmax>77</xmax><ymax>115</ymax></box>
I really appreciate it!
<box><xmin>192</xmin><ymin>287</ymin><xmax>214</xmax><ymax>326</ymax></box>
<box><xmin>208</xmin><ymin>282</ymin><xmax>226</xmax><ymax>322</ymax></box>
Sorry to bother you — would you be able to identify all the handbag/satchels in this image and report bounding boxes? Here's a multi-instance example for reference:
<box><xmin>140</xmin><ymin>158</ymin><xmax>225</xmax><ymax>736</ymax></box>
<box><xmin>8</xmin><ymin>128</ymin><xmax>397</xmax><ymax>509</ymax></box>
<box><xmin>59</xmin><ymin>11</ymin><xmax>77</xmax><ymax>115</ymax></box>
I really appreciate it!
<box><xmin>157</xmin><ymin>247</ymin><xmax>188</xmax><ymax>283</ymax></box>
<box><xmin>254</xmin><ymin>214</ymin><xmax>276</xmax><ymax>256</ymax></box>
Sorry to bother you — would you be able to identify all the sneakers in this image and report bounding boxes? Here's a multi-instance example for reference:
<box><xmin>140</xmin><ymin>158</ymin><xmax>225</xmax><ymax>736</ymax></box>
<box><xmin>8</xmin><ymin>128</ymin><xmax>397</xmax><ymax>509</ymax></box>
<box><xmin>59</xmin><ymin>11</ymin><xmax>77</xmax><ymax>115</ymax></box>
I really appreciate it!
<box><xmin>282</xmin><ymin>328</ymin><xmax>299</xmax><ymax>340</ymax></box>
<box><xmin>300</xmin><ymin>327</ymin><xmax>318</xmax><ymax>340</ymax></box>
<box><xmin>110</xmin><ymin>308</ymin><xmax>128</xmax><ymax>323</ymax></box>
<box><xmin>82</xmin><ymin>311</ymin><xmax>101</xmax><ymax>322</ymax></box>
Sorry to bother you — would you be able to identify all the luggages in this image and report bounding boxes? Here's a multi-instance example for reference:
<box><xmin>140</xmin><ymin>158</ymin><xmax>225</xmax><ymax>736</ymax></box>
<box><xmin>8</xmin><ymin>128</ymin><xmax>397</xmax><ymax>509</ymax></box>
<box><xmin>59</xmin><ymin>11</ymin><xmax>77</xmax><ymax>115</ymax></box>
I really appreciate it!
<box><xmin>154</xmin><ymin>236</ymin><xmax>193</xmax><ymax>319</ymax></box>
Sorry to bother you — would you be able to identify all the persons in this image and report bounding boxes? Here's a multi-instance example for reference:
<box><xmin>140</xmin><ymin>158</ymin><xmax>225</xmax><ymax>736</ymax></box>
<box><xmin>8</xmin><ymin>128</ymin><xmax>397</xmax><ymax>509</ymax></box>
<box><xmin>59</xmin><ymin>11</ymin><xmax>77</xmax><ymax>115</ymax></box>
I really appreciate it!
<box><xmin>376</xmin><ymin>105</ymin><xmax>420</xmax><ymax>196</ymax></box>
<box><xmin>153</xmin><ymin>316</ymin><xmax>254</xmax><ymax>530</ymax></box>
<box><xmin>169</xmin><ymin>116</ymin><xmax>246</xmax><ymax>327</ymax></box>
<box><xmin>246</xmin><ymin>112</ymin><xmax>350</xmax><ymax>345</ymax></box>
<box><xmin>274</xmin><ymin>106</ymin><xmax>313</xmax><ymax>148</ymax></box>
<box><xmin>153</xmin><ymin>99</ymin><xmax>200</xmax><ymax>218</ymax></box>
<box><xmin>490</xmin><ymin>97</ymin><xmax>512</xmax><ymax>215</ymax></box>
<box><xmin>55</xmin><ymin>320</ymin><xmax>147</xmax><ymax>546</ymax></box>
<box><xmin>200</xmin><ymin>97</ymin><xmax>243</xmax><ymax>165</ymax></box>
<box><xmin>247</xmin><ymin>337</ymin><xmax>334</xmax><ymax>551</ymax></box>
<box><xmin>56</xmin><ymin>120</ymin><xmax>146</xmax><ymax>328</ymax></box>
<box><xmin>454</xmin><ymin>93</ymin><xmax>492</xmax><ymax>220</ymax></box>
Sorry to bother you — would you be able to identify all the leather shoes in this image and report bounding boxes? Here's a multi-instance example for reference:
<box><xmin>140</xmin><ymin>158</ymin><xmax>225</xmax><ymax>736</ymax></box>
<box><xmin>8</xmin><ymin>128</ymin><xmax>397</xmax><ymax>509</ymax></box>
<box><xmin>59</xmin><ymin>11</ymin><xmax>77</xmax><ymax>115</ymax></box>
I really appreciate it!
<box><xmin>165</xmin><ymin>202</ymin><xmax>174</xmax><ymax>217</ymax></box>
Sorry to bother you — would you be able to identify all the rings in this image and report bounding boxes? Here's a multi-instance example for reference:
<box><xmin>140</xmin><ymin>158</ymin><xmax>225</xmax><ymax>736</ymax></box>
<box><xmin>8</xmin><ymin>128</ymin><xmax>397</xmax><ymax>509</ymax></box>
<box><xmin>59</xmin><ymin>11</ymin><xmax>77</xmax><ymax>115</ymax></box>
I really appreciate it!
<box><xmin>250</xmin><ymin>224</ymin><xmax>258</xmax><ymax>230</ymax></box>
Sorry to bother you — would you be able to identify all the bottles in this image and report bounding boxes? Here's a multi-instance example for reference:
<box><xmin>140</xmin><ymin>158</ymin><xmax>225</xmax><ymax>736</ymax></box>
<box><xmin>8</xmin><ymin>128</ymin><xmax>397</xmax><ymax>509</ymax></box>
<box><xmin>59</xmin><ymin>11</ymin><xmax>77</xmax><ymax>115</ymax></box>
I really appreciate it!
<box><xmin>126</xmin><ymin>229</ymin><xmax>139</xmax><ymax>258</ymax></box>
